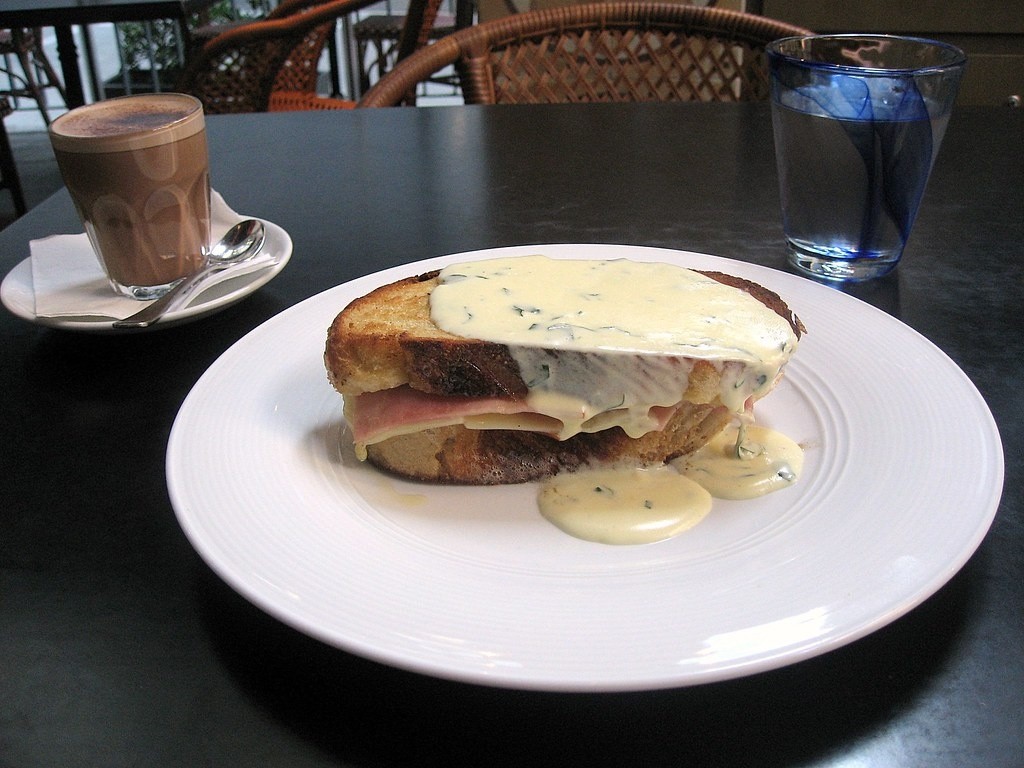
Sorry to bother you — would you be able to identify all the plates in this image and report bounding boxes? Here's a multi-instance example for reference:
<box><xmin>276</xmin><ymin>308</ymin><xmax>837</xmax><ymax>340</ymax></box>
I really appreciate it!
<box><xmin>164</xmin><ymin>240</ymin><xmax>1007</xmax><ymax>694</ymax></box>
<box><xmin>0</xmin><ymin>214</ymin><xmax>294</xmax><ymax>332</ymax></box>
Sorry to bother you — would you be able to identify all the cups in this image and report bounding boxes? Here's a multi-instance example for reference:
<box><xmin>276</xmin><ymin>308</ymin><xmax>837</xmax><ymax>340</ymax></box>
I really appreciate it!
<box><xmin>763</xmin><ymin>36</ymin><xmax>971</xmax><ymax>286</ymax></box>
<box><xmin>49</xmin><ymin>89</ymin><xmax>213</xmax><ymax>301</ymax></box>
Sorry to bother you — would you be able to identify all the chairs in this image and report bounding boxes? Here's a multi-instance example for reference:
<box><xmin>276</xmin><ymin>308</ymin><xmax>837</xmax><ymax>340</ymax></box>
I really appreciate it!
<box><xmin>0</xmin><ymin>0</ymin><xmax>877</xmax><ymax>220</ymax></box>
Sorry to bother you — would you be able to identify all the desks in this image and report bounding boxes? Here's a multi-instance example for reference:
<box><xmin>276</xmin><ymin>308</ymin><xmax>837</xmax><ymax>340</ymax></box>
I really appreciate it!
<box><xmin>8</xmin><ymin>103</ymin><xmax>1024</xmax><ymax>768</ymax></box>
<box><xmin>0</xmin><ymin>0</ymin><xmax>221</xmax><ymax>112</ymax></box>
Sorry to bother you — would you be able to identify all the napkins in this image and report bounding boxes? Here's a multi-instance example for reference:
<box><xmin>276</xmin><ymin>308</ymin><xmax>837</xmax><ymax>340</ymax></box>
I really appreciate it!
<box><xmin>26</xmin><ymin>185</ymin><xmax>280</xmax><ymax>323</ymax></box>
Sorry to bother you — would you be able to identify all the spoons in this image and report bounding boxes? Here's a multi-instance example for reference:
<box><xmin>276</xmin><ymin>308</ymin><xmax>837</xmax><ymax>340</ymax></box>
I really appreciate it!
<box><xmin>112</xmin><ymin>219</ymin><xmax>266</xmax><ymax>332</ymax></box>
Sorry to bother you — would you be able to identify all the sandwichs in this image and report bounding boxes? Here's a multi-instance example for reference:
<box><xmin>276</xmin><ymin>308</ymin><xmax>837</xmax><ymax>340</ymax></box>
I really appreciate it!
<box><xmin>323</xmin><ymin>252</ymin><xmax>806</xmax><ymax>485</ymax></box>
